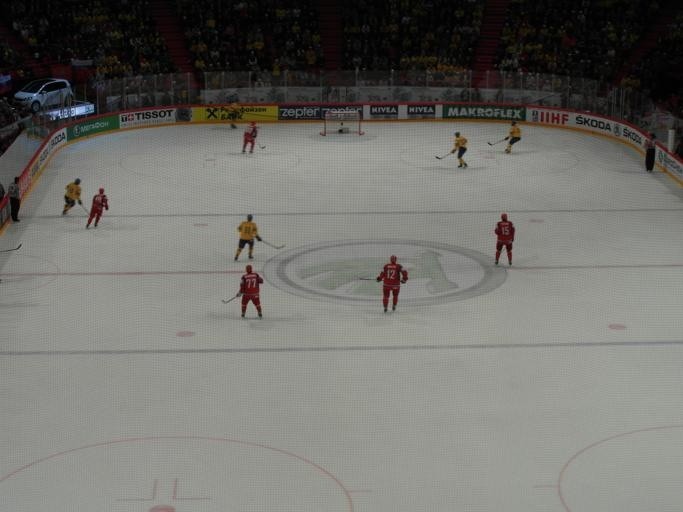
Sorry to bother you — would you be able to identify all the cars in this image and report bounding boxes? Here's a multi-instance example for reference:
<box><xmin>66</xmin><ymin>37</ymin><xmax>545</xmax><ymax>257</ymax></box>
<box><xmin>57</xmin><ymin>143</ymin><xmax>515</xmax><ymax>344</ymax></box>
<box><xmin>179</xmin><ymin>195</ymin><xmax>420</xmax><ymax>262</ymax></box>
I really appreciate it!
<box><xmin>12</xmin><ymin>78</ymin><xmax>74</xmax><ymax>112</ymax></box>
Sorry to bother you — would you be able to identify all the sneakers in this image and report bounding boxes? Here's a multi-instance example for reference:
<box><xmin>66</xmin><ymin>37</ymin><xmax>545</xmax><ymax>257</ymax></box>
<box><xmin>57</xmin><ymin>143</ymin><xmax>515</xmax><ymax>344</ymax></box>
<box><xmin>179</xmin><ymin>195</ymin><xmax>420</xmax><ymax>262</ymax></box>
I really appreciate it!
<box><xmin>457</xmin><ymin>162</ymin><xmax>467</xmax><ymax>168</ymax></box>
<box><xmin>240</xmin><ymin>312</ymin><xmax>245</xmax><ymax>317</ymax></box>
<box><xmin>257</xmin><ymin>312</ymin><xmax>262</xmax><ymax>317</ymax></box>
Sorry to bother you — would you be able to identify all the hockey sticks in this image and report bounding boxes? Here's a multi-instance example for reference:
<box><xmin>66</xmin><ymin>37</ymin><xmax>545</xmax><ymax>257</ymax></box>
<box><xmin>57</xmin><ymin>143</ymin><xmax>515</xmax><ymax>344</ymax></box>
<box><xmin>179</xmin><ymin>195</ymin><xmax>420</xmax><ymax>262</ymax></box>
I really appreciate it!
<box><xmin>221</xmin><ymin>296</ymin><xmax>237</xmax><ymax>304</ymax></box>
<box><xmin>253</xmin><ymin>236</ymin><xmax>285</xmax><ymax>249</ymax></box>
<box><xmin>0</xmin><ymin>243</ymin><xmax>21</xmax><ymax>252</ymax></box>
<box><xmin>359</xmin><ymin>276</ymin><xmax>402</xmax><ymax>282</ymax></box>
<box><xmin>487</xmin><ymin>138</ymin><xmax>506</xmax><ymax>146</ymax></box>
<box><xmin>255</xmin><ymin>137</ymin><xmax>266</xmax><ymax>149</ymax></box>
<box><xmin>435</xmin><ymin>152</ymin><xmax>452</xmax><ymax>160</ymax></box>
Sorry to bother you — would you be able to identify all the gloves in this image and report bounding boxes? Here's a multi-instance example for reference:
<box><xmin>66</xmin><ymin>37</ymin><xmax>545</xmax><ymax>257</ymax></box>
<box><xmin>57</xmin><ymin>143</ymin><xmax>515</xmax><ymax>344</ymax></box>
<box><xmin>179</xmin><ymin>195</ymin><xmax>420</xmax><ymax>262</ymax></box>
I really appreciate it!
<box><xmin>375</xmin><ymin>278</ymin><xmax>381</xmax><ymax>282</ymax></box>
<box><xmin>257</xmin><ymin>237</ymin><xmax>262</xmax><ymax>241</ymax></box>
<box><xmin>400</xmin><ymin>279</ymin><xmax>406</xmax><ymax>283</ymax></box>
<box><xmin>235</xmin><ymin>292</ymin><xmax>241</xmax><ymax>298</ymax></box>
<box><xmin>77</xmin><ymin>199</ymin><xmax>81</xmax><ymax>205</ymax></box>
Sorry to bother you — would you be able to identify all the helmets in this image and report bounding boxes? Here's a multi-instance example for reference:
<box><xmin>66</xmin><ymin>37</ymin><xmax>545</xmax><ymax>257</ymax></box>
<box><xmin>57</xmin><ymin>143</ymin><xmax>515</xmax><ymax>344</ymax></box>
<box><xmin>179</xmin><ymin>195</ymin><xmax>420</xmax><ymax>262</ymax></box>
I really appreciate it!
<box><xmin>98</xmin><ymin>186</ymin><xmax>104</xmax><ymax>195</ymax></box>
<box><xmin>389</xmin><ymin>255</ymin><xmax>396</xmax><ymax>263</ymax></box>
<box><xmin>453</xmin><ymin>131</ymin><xmax>460</xmax><ymax>138</ymax></box>
<box><xmin>73</xmin><ymin>178</ymin><xmax>80</xmax><ymax>185</ymax></box>
<box><xmin>501</xmin><ymin>213</ymin><xmax>507</xmax><ymax>222</ymax></box>
<box><xmin>245</xmin><ymin>264</ymin><xmax>253</xmax><ymax>274</ymax></box>
<box><xmin>246</xmin><ymin>214</ymin><xmax>252</xmax><ymax>222</ymax></box>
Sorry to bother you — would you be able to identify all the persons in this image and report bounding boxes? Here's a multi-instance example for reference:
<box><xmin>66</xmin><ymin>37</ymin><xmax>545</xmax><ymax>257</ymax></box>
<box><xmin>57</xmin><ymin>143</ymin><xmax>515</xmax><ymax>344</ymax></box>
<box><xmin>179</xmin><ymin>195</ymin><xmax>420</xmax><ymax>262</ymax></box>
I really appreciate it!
<box><xmin>449</xmin><ymin>130</ymin><xmax>469</xmax><ymax>168</ymax></box>
<box><xmin>8</xmin><ymin>175</ymin><xmax>22</xmax><ymax>222</ymax></box>
<box><xmin>233</xmin><ymin>213</ymin><xmax>263</xmax><ymax>262</ymax></box>
<box><xmin>61</xmin><ymin>178</ymin><xmax>83</xmax><ymax>216</ymax></box>
<box><xmin>376</xmin><ymin>255</ymin><xmax>408</xmax><ymax>313</ymax></box>
<box><xmin>493</xmin><ymin>212</ymin><xmax>516</xmax><ymax>267</ymax></box>
<box><xmin>85</xmin><ymin>186</ymin><xmax>109</xmax><ymax>230</ymax></box>
<box><xmin>642</xmin><ymin>132</ymin><xmax>658</xmax><ymax>172</ymax></box>
<box><xmin>0</xmin><ymin>0</ymin><xmax>682</xmax><ymax>163</ymax></box>
<box><xmin>235</xmin><ymin>264</ymin><xmax>266</xmax><ymax>319</ymax></box>
